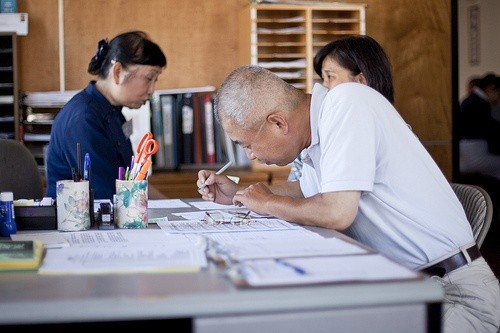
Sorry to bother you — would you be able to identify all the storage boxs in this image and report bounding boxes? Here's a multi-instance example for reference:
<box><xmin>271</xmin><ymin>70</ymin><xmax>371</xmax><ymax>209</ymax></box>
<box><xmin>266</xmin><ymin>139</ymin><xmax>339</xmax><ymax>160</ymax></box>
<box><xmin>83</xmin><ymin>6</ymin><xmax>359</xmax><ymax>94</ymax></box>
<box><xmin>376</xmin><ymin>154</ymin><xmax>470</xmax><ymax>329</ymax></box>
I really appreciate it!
<box><xmin>13</xmin><ymin>205</ymin><xmax>56</xmax><ymax>231</ymax></box>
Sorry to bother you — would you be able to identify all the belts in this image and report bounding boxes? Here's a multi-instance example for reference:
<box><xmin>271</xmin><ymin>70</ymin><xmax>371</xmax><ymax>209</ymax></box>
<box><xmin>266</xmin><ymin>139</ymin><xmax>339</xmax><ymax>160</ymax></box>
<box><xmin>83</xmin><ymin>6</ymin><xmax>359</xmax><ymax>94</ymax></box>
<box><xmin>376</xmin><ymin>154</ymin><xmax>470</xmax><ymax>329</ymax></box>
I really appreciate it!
<box><xmin>418</xmin><ymin>244</ymin><xmax>483</xmax><ymax>277</ymax></box>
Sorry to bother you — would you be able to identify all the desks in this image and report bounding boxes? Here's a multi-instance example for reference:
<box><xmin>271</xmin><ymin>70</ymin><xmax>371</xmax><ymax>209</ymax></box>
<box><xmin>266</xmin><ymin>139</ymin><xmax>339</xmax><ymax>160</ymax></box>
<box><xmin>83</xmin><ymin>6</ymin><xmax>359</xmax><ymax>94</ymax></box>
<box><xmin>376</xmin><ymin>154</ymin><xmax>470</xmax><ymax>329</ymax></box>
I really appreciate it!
<box><xmin>0</xmin><ymin>198</ymin><xmax>445</xmax><ymax>333</ymax></box>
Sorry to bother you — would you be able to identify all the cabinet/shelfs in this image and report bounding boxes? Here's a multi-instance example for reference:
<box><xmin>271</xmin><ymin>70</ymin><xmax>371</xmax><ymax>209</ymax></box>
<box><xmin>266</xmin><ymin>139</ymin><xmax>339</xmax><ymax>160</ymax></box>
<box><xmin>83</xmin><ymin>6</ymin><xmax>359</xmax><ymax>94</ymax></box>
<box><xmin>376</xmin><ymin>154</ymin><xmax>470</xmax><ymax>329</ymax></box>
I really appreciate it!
<box><xmin>238</xmin><ymin>3</ymin><xmax>366</xmax><ymax>96</ymax></box>
<box><xmin>0</xmin><ymin>32</ymin><xmax>22</xmax><ymax>141</ymax></box>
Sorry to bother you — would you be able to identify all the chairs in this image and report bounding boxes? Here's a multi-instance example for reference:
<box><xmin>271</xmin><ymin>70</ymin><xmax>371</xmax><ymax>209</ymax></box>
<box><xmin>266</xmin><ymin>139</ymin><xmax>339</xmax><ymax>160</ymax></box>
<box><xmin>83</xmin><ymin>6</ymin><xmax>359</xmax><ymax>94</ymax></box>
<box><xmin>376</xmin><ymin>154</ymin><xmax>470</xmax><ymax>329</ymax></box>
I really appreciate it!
<box><xmin>0</xmin><ymin>139</ymin><xmax>44</xmax><ymax>200</ymax></box>
<box><xmin>447</xmin><ymin>183</ymin><xmax>493</xmax><ymax>251</ymax></box>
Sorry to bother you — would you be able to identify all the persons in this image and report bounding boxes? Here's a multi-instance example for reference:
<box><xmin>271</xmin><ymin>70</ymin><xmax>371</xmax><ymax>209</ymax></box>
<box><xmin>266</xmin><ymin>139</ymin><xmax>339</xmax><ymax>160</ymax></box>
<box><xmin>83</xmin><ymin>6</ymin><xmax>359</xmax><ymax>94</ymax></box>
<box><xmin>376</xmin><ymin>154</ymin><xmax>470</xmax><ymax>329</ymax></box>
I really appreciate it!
<box><xmin>197</xmin><ymin>66</ymin><xmax>500</xmax><ymax>333</ymax></box>
<box><xmin>46</xmin><ymin>31</ymin><xmax>167</xmax><ymax>201</ymax></box>
<box><xmin>313</xmin><ymin>35</ymin><xmax>394</xmax><ymax>105</ymax></box>
<box><xmin>458</xmin><ymin>72</ymin><xmax>500</xmax><ymax>156</ymax></box>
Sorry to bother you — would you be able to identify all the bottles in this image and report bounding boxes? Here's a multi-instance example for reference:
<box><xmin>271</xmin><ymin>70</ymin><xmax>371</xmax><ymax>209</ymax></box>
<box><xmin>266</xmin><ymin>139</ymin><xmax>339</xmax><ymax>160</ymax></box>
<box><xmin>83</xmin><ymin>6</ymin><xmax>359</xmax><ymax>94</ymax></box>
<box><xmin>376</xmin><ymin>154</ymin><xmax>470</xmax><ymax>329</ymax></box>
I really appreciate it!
<box><xmin>0</xmin><ymin>191</ymin><xmax>16</xmax><ymax>237</ymax></box>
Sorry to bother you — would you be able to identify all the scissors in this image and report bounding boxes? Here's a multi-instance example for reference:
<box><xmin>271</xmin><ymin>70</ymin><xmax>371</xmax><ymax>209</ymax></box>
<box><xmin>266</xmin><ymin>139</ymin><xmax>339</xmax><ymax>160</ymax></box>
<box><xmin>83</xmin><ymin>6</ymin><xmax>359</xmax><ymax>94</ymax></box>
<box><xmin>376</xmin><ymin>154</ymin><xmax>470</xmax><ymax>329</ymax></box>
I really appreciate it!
<box><xmin>130</xmin><ymin>132</ymin><xmax>157</xmax><ymax>180</ymax></box>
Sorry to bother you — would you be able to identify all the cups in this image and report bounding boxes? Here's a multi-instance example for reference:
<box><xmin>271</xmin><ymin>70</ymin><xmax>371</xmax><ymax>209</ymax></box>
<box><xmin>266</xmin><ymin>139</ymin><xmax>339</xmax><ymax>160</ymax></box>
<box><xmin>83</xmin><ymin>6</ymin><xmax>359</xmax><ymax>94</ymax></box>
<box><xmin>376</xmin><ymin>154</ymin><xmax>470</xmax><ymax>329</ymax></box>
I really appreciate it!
<box><xmin>56</xmin><ymin>180</ymin><xmax>91</xmax><ymax>232</ymax></box>
<box><xmin>116</xmin><ymin>179</ymin><xmax>148</xmax><ymax>229</ymax></box>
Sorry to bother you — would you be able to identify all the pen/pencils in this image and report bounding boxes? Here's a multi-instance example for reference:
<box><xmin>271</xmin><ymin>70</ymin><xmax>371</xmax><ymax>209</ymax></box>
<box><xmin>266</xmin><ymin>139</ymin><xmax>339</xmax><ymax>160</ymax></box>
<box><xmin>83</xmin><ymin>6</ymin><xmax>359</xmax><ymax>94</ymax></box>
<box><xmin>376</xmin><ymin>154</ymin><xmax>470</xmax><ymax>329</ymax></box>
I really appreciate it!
<box><xmin>276</xmin><ymin>258</ymin><xmax>304</xmax><ymax>275</ymax></box>
<box><xmin>70</xmin><ymin>166</ymin><xmax>75</xmax><ymax>178</ymax></box>
<box><xmin>119</xmin><ymin>156</ymin><xmax>149</xmax><ymax>181</ymax></box>
<box><xmin>84</xmin><ymin>153</ymin><xmax>91</xmax><ymax>180</ymax></box>
<box><xmin>76</xmin><ymin>142</ymin><xmax>81</xmax><ymax>180</ymax></box>
<box><xmin>197</xmin><ymin>160</ymin><xmax>233</xmax><ymax>191</ymax></box>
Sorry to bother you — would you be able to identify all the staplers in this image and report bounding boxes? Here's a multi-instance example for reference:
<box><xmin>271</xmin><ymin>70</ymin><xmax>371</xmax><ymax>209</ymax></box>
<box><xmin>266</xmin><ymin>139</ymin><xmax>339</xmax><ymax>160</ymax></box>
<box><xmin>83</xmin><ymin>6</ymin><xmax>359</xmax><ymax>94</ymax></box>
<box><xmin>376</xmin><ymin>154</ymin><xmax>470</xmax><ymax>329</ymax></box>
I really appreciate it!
<box><xmin>98</xmin><ymin>202</ymin><xmax>115</xmax><ymax>230</ymax></box>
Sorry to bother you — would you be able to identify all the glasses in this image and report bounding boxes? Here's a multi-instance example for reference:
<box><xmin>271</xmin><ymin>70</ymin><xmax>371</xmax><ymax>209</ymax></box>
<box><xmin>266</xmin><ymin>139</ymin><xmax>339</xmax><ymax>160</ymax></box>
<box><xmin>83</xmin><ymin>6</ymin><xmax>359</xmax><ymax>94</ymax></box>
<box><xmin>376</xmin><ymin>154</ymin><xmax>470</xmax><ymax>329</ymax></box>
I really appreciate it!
<box><xmin>198</xmin><ymin>210</ymin><xmax>254</xmax><ymax>228</ymax></box>
